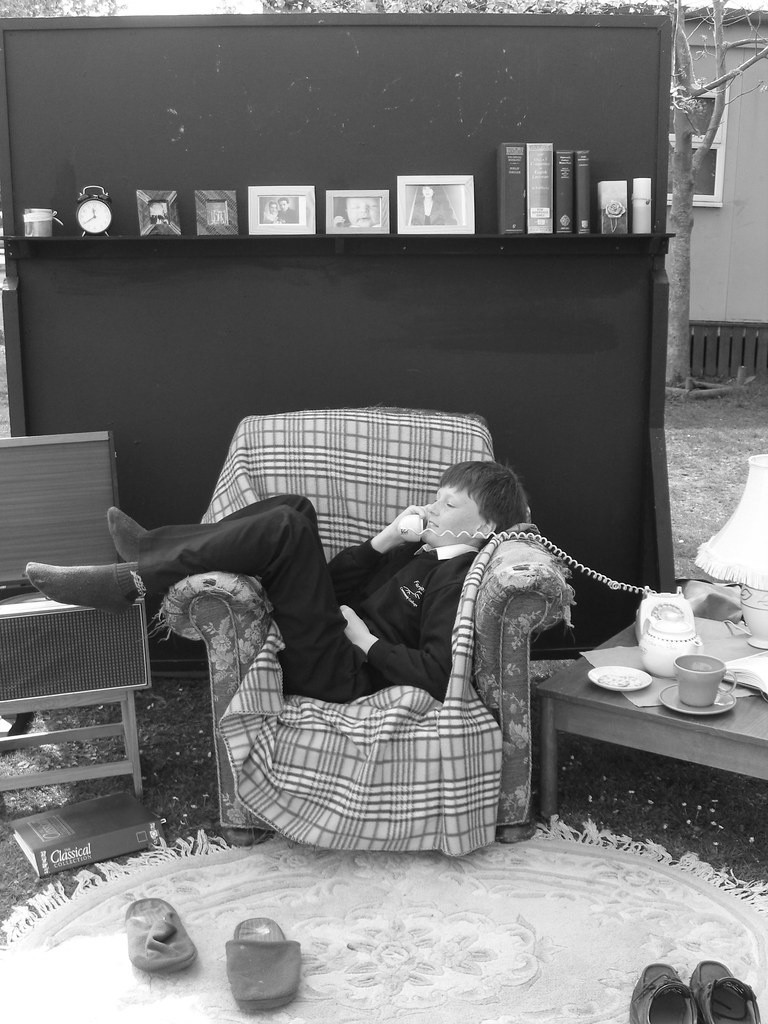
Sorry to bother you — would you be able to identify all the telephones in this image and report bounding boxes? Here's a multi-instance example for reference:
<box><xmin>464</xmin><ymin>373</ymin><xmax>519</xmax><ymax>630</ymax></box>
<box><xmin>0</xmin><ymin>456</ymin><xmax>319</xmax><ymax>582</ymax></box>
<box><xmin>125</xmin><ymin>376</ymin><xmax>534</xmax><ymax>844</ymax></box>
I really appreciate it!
<box><xmin>397</xmin><ymin>514</ymin><xmax>426</xmax><ymax>543</ymax></box>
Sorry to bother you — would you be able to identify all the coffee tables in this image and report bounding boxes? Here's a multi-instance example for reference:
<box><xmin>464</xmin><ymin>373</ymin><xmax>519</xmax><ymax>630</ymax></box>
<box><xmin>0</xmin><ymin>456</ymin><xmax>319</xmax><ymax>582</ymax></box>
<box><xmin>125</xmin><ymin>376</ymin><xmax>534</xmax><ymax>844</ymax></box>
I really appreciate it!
<box><xmin>536</xmin><ymin>598</ymin><xmax>768</xmax><ymax>854</ymax></box>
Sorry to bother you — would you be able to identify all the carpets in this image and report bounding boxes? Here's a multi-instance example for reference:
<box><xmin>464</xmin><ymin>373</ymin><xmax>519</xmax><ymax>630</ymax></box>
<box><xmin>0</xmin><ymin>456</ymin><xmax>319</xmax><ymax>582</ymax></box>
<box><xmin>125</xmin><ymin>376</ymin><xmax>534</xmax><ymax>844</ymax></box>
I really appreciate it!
<box><xmin>0</xmin><ymin>814</ymin><xmax>768</xmax><ymax>1023</ymax></box>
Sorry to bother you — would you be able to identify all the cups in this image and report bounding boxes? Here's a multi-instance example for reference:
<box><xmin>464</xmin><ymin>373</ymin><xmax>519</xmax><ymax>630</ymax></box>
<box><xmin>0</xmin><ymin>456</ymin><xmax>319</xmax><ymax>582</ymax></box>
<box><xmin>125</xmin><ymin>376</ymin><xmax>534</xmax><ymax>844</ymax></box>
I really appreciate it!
<box><xmin>23</xmin><ymin>207</ymin><xmax>53</xmax><ymax>236</ymax></box>
<box><xmin>675</xmin><ymin>658</ymin><xmax>737</xmax><ymax>707</ymax></box>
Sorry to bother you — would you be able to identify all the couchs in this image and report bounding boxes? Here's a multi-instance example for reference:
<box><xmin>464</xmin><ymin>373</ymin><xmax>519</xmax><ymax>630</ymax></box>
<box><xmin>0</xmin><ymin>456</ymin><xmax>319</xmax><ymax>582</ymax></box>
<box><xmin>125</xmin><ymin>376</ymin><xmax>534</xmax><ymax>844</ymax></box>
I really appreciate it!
<box><xmin>164</xmin><ymin>408</ymin><xmax>574</xmax><ymax>843</ymax></box>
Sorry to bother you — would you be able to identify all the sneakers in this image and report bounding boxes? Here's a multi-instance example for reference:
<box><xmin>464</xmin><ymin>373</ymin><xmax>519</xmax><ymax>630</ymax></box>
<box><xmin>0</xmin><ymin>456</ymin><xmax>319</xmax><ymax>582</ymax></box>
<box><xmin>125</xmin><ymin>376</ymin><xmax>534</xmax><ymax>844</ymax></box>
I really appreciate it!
<box><xmin>690</xmin><ymin>960</ymin><xmax>761</xmax><ymax>1024</ymax></box>
<box><xmin>629</xmin><ymin>963</ymin><xmax>697</xmax><ymax>1024</ymax></box>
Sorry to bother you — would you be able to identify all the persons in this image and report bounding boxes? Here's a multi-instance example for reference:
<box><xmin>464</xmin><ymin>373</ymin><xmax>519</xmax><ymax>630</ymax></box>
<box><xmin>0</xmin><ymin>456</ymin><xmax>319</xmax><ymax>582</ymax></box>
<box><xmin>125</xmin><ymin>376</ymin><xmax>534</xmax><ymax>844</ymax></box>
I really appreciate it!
<box><xmin>264</xmin><ymin>197</ymin><xmax>297</xmax><ymax>223</ymax></box>
<box><xmin>207</xmin><ymin>209</ymin><xmax>226</xmax><ymax>222</ymax></box>
<box><xmin>333</xmin><ymin>197</ymin><xmax>380</xmax><ymax>227</ymax></box>
<box><xmin>413</xmin><ymin>186</ymin><xmax>445</xmax><ymax>225</ymax></box>
<box><xmin>26</xmin><ymin>460</ymin><xmax>526</xmax><ymax>705</ymax></box>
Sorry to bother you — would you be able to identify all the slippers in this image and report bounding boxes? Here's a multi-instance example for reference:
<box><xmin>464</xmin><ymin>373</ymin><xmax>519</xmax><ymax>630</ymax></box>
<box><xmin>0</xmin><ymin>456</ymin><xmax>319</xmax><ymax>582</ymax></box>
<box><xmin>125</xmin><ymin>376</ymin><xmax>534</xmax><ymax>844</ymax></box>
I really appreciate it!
<box><xmin>126</xmin><ymin>898</ymin><xmax>197</xmax><ymax>973</ymax></box>
<box><xmin>226</xmin><ymin>917</ymin><xmax>302</xmax><ymax>1008</ymax></box>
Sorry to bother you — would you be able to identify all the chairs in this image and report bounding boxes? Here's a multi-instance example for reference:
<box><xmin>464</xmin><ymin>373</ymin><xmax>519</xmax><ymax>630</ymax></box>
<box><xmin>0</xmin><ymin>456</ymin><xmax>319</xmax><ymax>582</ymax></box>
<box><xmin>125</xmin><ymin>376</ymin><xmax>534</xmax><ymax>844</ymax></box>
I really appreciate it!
<box><xmin>0</xmin><ymin>431</ymin><xmax>153</xmax><ymax>821</ymax></box>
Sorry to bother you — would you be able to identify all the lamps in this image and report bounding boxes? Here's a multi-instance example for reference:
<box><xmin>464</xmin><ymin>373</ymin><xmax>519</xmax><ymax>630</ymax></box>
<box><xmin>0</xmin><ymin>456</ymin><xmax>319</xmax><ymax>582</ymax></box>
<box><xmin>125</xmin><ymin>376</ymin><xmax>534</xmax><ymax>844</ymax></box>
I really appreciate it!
<box><xmin>694</xmin><ymin>453</ymin><xmax>768</xmax><ymax>651</ymax></box>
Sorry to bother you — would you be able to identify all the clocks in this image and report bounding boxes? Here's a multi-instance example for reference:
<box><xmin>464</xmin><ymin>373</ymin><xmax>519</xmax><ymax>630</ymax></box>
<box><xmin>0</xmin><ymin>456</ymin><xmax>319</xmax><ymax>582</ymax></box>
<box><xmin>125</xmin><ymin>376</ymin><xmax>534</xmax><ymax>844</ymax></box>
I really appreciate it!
<box><xmin>74</xmin><ymin>186</ymin><xmax>114</xmax><ymax>237</ymax></box>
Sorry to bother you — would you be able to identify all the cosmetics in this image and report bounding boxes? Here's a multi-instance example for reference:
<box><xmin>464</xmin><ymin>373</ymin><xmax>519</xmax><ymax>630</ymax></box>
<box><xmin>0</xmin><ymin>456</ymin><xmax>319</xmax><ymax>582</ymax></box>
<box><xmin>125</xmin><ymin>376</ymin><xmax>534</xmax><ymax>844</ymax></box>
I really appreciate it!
<box><xmin>496</xmin><ymin>142</ymin><xmax>653</xmax><ymax>234</ymax></box>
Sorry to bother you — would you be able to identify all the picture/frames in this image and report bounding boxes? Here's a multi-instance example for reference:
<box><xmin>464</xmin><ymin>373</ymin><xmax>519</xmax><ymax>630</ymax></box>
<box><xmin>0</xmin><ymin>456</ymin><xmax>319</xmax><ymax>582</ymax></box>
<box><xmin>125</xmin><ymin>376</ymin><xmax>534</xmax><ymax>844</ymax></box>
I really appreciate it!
<box><xmin>325</xmin><ymin>189</ymin><xmax>390</xmax><ymax>235</ymax></box>
<box><xmin>397</xmin><ymin>174</ymin><xmax>476</xmax><ymax>235</ymax></box>
<box><xmin>248</xmin><ymin>185</ymin><xmax>316</xmax><ymax>235</ymax></box>
<box><xmin>136</xmin><ymin>190</ymin><xmax>182</xmax><ymax>237</ymax></box>
<box><xmin>195</xmin><ymin>189</ymin><xmax>239</xmax><ymax>237</ymax></box>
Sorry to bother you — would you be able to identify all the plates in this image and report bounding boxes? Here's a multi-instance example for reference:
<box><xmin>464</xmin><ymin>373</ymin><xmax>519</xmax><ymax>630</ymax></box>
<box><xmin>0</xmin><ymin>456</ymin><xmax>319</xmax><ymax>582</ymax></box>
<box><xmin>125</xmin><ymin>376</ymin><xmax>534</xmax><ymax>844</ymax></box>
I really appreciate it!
<box><xmin>587</xmin><ymin>666</ymin><xmax>652</xmax><ymax>692</ymax></box>
<box><xmin>659</xmin><ymin>684</ymin><xmax>737</xmax><ymax>715</ymax></box>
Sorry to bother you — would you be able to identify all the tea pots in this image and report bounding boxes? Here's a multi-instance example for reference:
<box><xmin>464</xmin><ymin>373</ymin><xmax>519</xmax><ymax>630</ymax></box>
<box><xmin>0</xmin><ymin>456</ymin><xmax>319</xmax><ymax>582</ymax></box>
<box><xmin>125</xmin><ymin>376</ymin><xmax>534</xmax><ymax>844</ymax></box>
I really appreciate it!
<box><xmin>638</xmin><ymin>612</ymin><xmax>704</xmax><ymax>678</ymax></box>
